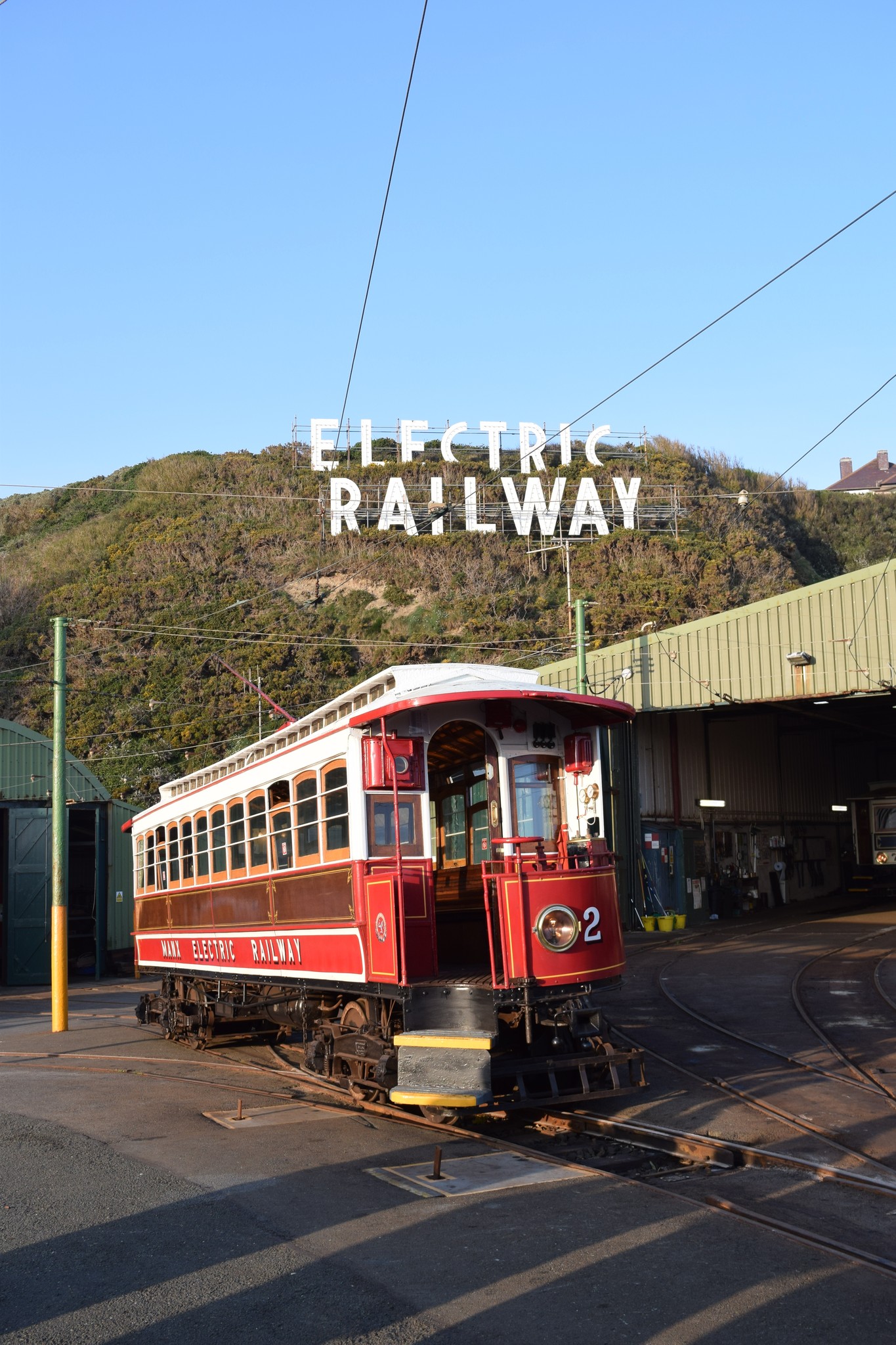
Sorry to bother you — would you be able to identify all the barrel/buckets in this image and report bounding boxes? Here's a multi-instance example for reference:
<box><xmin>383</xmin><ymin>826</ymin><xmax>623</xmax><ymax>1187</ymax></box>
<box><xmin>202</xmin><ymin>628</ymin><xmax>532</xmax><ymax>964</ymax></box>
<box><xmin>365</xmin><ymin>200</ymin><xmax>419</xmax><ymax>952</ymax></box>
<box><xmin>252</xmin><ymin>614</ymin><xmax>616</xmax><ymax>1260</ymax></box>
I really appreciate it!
<box><xmin>656</xmin><ymin>916</ymin><xmax>674</xmax><ymax>932</ymax></box>
<box><xmin>674</xmin><ymin>914</ymin><xmax>686</xmax><ymax>929</ymax></box>
<box><xmin>640</xmin><ymin>916</ymin><xmax>656</xmax><ymax>931</ymax></box>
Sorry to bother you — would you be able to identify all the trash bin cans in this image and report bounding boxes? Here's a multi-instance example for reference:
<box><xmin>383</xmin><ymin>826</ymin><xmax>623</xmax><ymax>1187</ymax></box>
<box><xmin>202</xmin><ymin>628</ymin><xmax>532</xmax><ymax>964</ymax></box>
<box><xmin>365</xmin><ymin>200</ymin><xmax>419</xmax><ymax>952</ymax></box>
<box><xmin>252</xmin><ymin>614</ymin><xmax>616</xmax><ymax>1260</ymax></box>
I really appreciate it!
<box><xmin>708</xmin><ymin>884</ymin><xmax>728</xmax><ymax>918</ymax></box>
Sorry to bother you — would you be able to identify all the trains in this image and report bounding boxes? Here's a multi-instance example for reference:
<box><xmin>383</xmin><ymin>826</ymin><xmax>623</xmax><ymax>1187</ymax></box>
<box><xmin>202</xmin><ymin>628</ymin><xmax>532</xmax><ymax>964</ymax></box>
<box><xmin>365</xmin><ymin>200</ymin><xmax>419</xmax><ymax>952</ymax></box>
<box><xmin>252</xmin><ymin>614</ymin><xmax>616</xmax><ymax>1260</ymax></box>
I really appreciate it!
<box><xmin>121</xmin><ymin>661</ymin><xmax>656</xmax><ymax>1127</ymax></box>
<box><xmin>843</xmin><ymin>781</ymin><xmax>896</xmax><ymax>896</ymax></box>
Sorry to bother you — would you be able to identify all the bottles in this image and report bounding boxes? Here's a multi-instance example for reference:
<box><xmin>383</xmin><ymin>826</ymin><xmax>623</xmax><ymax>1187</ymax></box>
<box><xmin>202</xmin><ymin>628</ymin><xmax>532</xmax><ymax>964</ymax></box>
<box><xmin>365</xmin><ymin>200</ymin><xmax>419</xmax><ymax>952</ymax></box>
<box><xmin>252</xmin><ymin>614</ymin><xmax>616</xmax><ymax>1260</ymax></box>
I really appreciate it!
<box><xmin>772</xmin><ymin>836</ymin><xmax>777</xmax><ymax>847</ymax></box>
<box><xmin>768</xmin><ymin>837</ymin><xmax>773</xmax><ymax>847</ymax></box>
<box><xmin>738</xmin><ymin>867</ymin><xmax>742</xmax><ymax>878</ymax></box>
<box><xmin>720</xmin><ymin>865</ymin><xmax>723</xmax><ymax>875</ymax></box>
<box><xmin>780</xmin><ymin>836</ymin><xmax>783</xmax><ymax>847</ymax></box>
<box><xmin>783</xmin><ymin>836</ymin><xmax>785</xmax><ymax>847</ymax></box>
<box><xmin>777</xmin><ymin>836</ymin><xmax>780</xmax><ymax>847</ymax></box>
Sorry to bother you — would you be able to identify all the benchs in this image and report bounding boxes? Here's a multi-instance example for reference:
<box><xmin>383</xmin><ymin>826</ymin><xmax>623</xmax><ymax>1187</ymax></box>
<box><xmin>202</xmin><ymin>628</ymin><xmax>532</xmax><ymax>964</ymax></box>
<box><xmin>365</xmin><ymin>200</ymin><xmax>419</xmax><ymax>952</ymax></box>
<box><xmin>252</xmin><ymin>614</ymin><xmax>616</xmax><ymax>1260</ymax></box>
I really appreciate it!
<box><xmin>432</xmin><ymin>861</ymin><xmax>494</xmax><ymax>925</ymax></box>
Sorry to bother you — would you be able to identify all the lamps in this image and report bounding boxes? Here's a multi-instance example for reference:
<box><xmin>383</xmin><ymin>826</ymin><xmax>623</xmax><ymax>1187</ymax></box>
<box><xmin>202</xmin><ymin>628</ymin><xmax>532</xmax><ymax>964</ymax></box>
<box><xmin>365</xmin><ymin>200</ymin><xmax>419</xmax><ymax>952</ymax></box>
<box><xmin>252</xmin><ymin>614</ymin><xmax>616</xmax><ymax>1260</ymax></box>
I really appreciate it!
<box><xmin>696</xmin><ymin>799</ymin><xmax>726</xmax><ymax>808</ymax></box>
<box><xmin>786</xmin><ymin>651</ymin><xmax>812</xmax><ymax>668</ymax></box>
<box><xmin>832</xmin><ymin>805</ymin><xmax>847</xmax><ymax>811</ymax></box>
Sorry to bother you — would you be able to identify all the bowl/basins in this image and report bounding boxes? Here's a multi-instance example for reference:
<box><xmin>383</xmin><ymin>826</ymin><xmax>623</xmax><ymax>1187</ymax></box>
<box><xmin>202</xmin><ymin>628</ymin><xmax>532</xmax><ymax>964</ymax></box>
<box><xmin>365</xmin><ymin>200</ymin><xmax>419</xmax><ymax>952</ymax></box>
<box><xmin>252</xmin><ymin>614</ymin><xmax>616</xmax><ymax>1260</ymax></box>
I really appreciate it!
<box><xmin>743</xmin><ymin>875</ymin><xmax>748</xmax><ymax>878</ymax></box>
